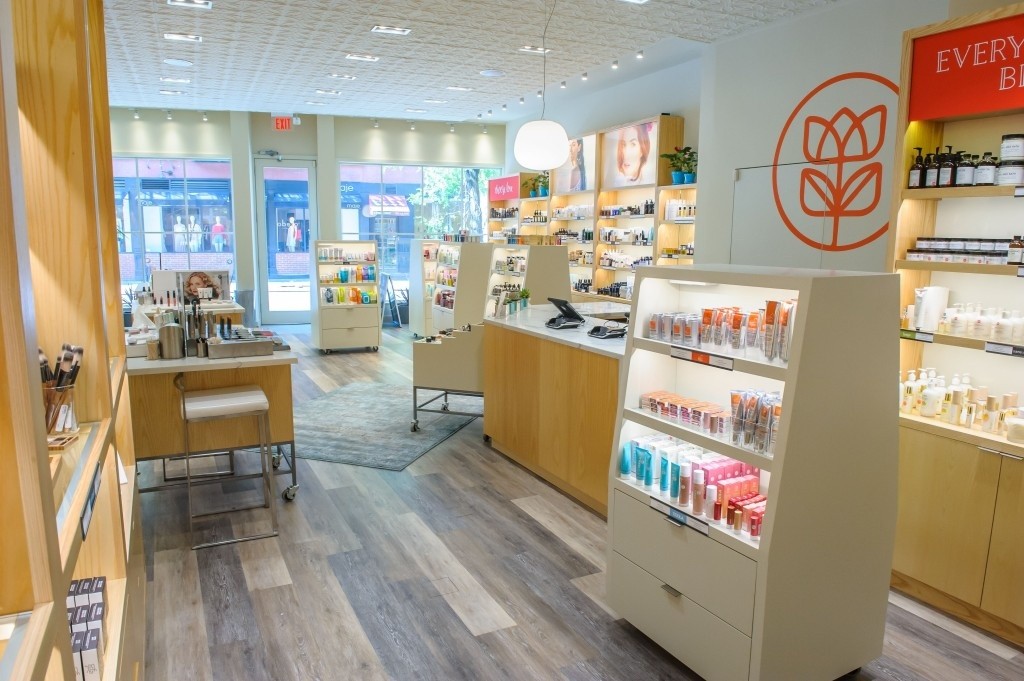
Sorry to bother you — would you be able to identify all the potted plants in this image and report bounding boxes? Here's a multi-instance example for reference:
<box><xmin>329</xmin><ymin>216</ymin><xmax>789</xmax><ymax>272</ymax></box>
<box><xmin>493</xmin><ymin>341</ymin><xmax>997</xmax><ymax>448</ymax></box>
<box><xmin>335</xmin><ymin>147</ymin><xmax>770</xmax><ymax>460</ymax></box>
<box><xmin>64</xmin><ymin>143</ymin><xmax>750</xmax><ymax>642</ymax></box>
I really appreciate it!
<box><xmin>495</xmin><ymin>288</ymin><xmax>531</xmax><ymax>319</ymax></box>
<box><xmin>521</xmin><ymin>170</ymin><xmax>549</xmax><ymax>198</ymax></box>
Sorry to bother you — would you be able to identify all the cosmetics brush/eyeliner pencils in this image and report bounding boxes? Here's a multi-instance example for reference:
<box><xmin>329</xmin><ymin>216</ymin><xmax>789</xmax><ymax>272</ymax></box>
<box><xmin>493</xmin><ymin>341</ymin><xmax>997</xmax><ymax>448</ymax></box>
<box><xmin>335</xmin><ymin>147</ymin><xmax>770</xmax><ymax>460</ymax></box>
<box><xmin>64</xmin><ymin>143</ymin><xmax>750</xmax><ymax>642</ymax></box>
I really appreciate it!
<box><xmin>38</xmin><ymin>344</ymin><xmax>83</xmax><ymax>435</ymax></box>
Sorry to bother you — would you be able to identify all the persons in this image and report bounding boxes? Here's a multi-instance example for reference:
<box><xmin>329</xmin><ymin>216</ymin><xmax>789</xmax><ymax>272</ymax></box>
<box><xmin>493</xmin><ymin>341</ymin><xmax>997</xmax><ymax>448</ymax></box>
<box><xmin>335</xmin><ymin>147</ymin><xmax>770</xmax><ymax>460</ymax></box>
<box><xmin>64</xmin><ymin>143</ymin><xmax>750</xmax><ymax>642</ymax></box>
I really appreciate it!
<box><xmin>570</xmin><ymin>138</ymin><xmax>586</xmax><ymax>192</ymax></box>
<box><xmin>614</xmin><ymin>124</ymin><xmax>651</xmax><ymax>185</ymax></box>
<box><xmin>116</xmin><ymin>210</ymin><xmax>123</xmax><ymax>253</ymax></box>
<box><xmin>184</xmin><ymin>272</ymin><xmax>220</xmax><ymax>304</ymax></box>
<box><xmin>173</xmin><ymin>216</ymin><xmax>187</xmax><ymax>253</ymax></box>
<box><xmin>212</xmin><ymin>216</ymin><xmax>226</xmax><ymax>252</ymax></box>
<box><xmin>188</xmin><ymin>215</ymin><xmax>202</xmax><ymax>252</ymax></box>
<box><xmin>287</xmin><ymin>217</ymin><xmax>297</xmax><ymax>253</ymax></box>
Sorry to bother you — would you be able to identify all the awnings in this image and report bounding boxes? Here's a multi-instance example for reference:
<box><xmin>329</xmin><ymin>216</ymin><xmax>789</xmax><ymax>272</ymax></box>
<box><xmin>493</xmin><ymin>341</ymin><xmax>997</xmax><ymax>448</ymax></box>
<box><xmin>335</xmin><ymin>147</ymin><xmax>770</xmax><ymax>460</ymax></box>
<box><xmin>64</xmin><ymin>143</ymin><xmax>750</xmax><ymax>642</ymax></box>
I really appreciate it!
<box><xmin>370</xmin><ymin>194</ymin><xmax>410</xmax><ymax>217</ymax></box>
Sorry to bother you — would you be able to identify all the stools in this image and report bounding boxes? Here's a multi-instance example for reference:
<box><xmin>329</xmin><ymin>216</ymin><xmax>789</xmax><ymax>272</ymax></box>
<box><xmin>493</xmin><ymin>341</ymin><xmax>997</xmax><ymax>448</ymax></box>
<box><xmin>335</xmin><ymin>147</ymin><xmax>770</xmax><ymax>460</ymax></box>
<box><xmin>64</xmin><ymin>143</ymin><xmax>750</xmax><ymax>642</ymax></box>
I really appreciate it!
<box><xmin>174</xmin><ymin>371</ymin><xmax>280</xmax><ymax>550</ymax></box>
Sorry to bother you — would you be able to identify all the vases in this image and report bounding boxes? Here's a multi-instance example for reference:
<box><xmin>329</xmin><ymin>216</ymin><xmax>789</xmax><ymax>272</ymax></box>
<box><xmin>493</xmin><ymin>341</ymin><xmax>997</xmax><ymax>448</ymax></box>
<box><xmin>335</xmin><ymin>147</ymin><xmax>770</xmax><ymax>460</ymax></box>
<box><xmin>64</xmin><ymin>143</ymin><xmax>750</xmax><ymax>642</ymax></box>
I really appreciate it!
<box><xmin>659</xmin><ymin>145</ymin><xmax>698</xmax><ymax>185</ymax></box>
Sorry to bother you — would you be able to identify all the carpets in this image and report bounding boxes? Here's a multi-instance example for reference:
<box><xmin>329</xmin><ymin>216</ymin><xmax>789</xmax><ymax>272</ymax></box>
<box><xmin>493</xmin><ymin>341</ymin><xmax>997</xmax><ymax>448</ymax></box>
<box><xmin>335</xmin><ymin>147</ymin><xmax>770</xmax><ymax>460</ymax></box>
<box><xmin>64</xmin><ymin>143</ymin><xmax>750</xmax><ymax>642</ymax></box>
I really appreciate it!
<box><xmin>242</xmin><ymin>379</ymin><xmax>484</xmax><ymax>473</ymax></box>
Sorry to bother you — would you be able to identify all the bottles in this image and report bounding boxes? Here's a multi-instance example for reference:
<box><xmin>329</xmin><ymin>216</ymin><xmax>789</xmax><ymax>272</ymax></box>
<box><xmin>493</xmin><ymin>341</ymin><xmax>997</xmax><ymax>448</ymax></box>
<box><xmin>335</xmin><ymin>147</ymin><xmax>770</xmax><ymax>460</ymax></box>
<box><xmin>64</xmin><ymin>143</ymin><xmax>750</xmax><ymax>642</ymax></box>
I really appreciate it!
<box><xmin>955</xmin><ymin>133</ymin><xmax>1024</xmax><ymax>187</ymax></box>
<box><xmin>906</xmin><ymin>236</ymin><xmax>1024</xmax><ymax>265</ymax></box>
<box><xmin>972</xmin><ymin>308</ymin><xmax>1024</xmax><ymax>346</ymax></box>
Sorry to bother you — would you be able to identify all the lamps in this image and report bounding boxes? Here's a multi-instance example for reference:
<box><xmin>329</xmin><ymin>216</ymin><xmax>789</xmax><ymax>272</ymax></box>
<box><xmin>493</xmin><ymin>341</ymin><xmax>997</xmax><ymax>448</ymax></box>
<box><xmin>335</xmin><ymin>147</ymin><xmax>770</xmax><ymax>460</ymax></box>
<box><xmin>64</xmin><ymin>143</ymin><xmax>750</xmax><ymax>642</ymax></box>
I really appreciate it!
<box><xmin>514</xmin><ymin>0</ymin><xmax>572</xmax><ymax>170</ymax></box>
<box><xmin>373</xmin><ymin>51</ymin><xmax>644</xmax><ymax>135</ymax></box>
<box><xmin>134</xmin><ymin>109</ymin><xmax>208</xmax><ymax>121</ymax></box>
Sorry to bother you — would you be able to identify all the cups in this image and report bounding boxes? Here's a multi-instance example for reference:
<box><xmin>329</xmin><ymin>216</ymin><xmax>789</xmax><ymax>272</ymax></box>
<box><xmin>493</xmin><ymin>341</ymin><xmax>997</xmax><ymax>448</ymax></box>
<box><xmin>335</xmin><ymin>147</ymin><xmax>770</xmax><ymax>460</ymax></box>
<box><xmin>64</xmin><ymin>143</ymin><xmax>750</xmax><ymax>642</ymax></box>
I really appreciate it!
<box><xmin>43</xmin><ymin>384</ymin><xmax>79</xmax><ymax>435</ymax></box>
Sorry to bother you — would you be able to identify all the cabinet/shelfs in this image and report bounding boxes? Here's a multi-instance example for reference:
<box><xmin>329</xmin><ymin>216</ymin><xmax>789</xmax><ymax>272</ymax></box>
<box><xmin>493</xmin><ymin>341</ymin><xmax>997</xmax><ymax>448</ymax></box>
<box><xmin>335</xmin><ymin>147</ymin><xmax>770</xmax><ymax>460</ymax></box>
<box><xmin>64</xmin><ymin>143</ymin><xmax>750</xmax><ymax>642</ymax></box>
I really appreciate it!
<box><xmin>309</xmin><ymin>239</ymin><xmax>382</xmax><ymax>356</ymax></box>
<box><xmin>0</xmin><ymin>0</ymin><xmax>147</xmax><ymax>681</ymax></box>
<box><xmin>431</xmin><ymin>241</ymin><xmax>494</xmax><ymax>338</ymax></box>
<box><xmin>485</xmin><ymin>244</ymin><xmax>573</xmax><ymax>316</ymax></box>
<box><xmin>485</xmin><ymin>170</ymin><xmax>698</xmax><ymax>305</ymax></box>
<box><xmin>884</xmin><ymin>0</ymin><xmax>1024</xmax><ymax>652</ymax></box>
<box><xmin>606</xmin><ymin>261</ymin><xmax>902</xmax><ymax>681</ymax></box>
<box><xmin>409</xmin><ymin>238</ymin><xmax>444</xmax><ymax>340</ymax></box>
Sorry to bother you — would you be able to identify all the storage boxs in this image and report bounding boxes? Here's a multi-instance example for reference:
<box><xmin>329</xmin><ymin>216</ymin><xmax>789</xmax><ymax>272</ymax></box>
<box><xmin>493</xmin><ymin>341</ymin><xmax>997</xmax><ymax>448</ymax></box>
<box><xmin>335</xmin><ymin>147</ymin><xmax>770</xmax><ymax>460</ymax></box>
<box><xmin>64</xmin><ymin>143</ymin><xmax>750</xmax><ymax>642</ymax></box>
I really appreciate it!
<box><xmin>65</xmin><ymin>576</ymin><xmax>110</xmax><ymax>681</ymax></box>
<box><xmin>631</xmin><ymin>390</ymin><xmax>769</xmax><ymax>536</ymax></box>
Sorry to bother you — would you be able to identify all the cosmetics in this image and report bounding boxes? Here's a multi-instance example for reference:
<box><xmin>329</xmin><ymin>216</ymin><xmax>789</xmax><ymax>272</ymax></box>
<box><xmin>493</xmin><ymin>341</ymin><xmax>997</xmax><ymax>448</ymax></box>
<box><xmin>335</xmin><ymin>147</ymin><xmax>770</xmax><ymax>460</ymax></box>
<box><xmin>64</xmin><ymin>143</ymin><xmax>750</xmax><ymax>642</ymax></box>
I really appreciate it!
<box><xmin>143</xmin><ymin>286</ymin><xmax>282</xmax><ymax>346</ymax></box>
<box><xmin>897</xmin><ymin>367</ymin><xmax>1024</xmax><ymax>444</ymax></box>
<box><xmin>907</xmin><ymin>134</ymin><xmax>1024</xmax><ymax>189</ymax></box>
<box><xmin>906</xmin><ymin>235</ymin><xmax>1024</xmax><ymax>267</ymax></box>
<box><xmin>318</xmin><ymin>247</ymin><xmax>377</xmax><ymax>305</ymax></box>
<box><xmin>422</xmin><ymin>199</ymin><xmax>696</xmax><ymax>317</ymax></box>
<box><xmin>899</xmin><ymin>287</ymin><xmax>1024</xmax><ymax>348</ymax></box>
<box><xmin>618</xmin><ymin>296</ymin><xmax>799</xmax><ymax>542</ymax></box>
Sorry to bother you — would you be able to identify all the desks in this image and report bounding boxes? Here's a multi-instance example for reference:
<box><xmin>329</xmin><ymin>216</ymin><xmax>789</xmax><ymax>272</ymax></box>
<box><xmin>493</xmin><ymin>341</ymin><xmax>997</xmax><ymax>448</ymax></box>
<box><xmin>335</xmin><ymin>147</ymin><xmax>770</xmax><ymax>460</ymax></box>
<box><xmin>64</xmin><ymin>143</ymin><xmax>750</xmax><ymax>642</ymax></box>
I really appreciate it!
<box><xmin>121</xmin><ymin>330</ymin><xmax>299</xmax><ymax>503</ymax></box>
<box><xmin>130</xmin><ymin>298</ymin><xmax>246</xmax><ymax>326</ymax></box>
